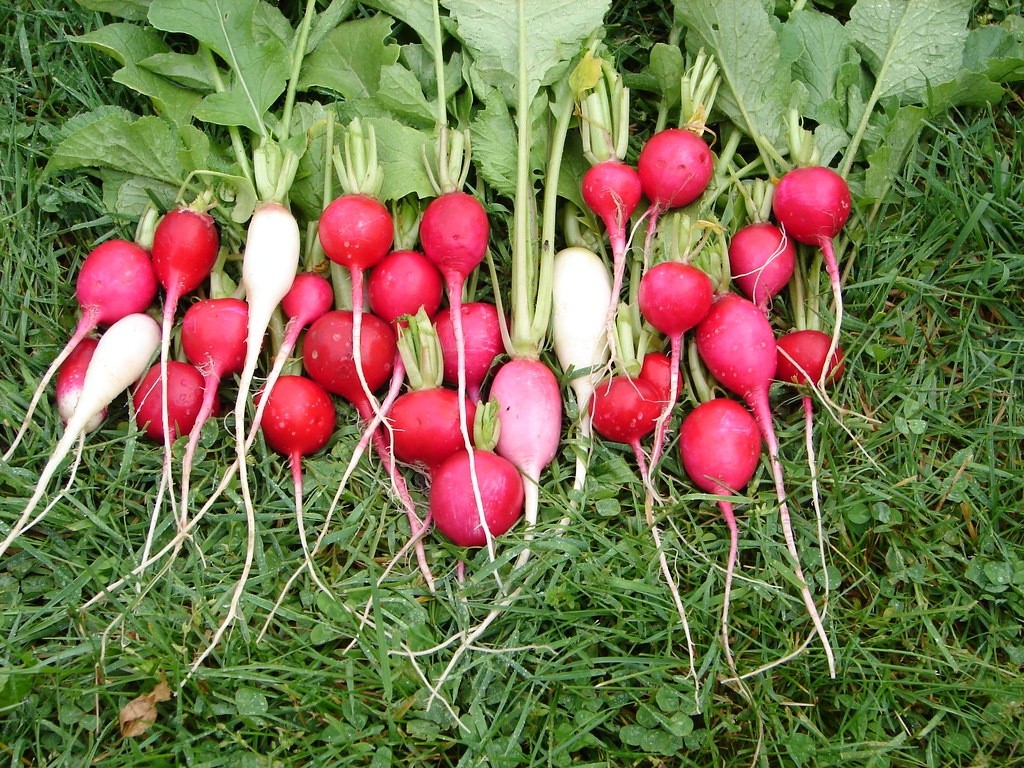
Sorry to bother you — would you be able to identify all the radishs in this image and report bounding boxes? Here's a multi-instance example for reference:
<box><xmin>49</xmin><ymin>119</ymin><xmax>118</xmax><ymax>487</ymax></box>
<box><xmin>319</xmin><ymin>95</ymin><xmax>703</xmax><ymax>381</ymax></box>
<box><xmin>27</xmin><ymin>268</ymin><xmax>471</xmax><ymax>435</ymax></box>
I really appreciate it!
<box><xmin>426</xmin><ymin>247</ymin><xmax>613</xmax><ymax>716</ymax></box>
<box><xmin>0</xmin><ymin>313</ymin><xmax>161</xmax><ymax>560</ymax></box>
<box><xmin>174</xmin><ymin>202</ymin><xmax>304</xmax><ymax>698</ymax></box>
